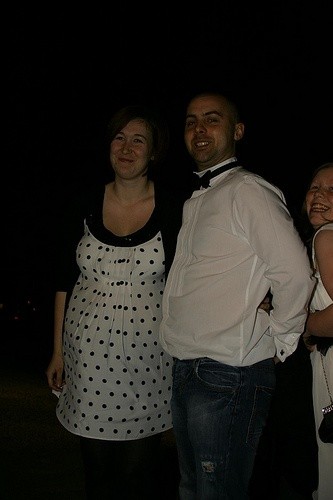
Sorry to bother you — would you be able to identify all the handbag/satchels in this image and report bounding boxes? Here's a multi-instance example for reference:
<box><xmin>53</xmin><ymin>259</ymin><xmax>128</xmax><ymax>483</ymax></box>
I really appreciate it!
<box><xmin>318</xmin><ymin>348</ymin><xmax>333</xmax><ymax>444</ymax></box>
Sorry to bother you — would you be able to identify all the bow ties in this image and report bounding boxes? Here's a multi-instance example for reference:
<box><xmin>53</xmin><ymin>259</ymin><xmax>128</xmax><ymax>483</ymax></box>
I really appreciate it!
<box><xmin>190</xmin><ymin>161</ymin><xmax>241</xmax><ymax>191</ymax></box>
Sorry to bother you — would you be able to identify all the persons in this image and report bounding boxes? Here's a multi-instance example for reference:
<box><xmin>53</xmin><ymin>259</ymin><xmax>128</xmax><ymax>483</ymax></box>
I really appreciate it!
<box><xmin>44</xmin><ymin>103</ymin><xmax>189</xmax><ymax>499</ymax></box>
<box><xmin>161</xmin><ymin>94</ymin><xmax>319</xmax><ymax>500</ymax></box>
<box><xmin>256</xmin><ymin>161</ymin><xmax>333</xmax><ymax>499</ymax></box>
